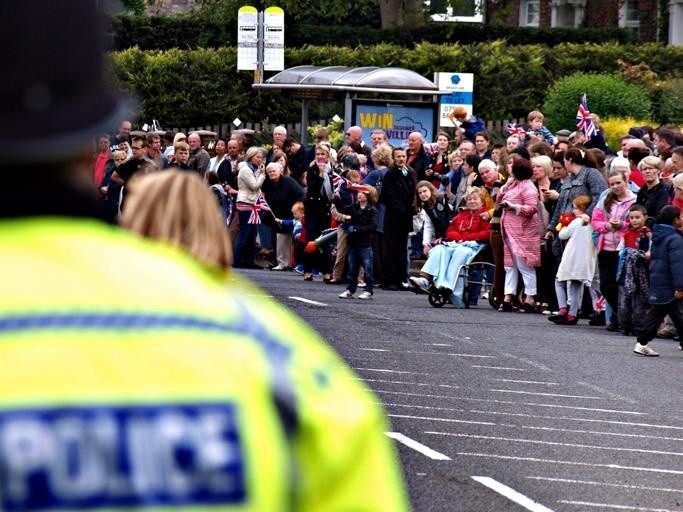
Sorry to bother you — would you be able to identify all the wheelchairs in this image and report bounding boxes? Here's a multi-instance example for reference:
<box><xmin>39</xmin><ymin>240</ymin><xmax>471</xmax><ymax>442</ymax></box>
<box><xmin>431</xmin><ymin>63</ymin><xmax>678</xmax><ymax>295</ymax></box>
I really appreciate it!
<box><xmin>428</xmin><ymin>233</ymin><xmax>500</xmax><ymax>309</ymax></box>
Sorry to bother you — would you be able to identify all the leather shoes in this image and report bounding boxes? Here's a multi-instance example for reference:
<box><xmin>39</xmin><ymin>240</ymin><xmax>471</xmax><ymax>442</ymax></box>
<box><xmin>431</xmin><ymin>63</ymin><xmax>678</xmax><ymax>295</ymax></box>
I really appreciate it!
<box><xmin>272</xmin><ymin>265</ymin><xmax>293</xmax><ymax>270</ymax></box>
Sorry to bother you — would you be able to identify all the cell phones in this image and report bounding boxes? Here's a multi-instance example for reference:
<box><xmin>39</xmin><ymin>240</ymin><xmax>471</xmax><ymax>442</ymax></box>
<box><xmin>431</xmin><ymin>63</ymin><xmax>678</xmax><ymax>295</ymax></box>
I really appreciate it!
<box><xmin>541</xmin><ymin>189</ymin><xmax>549</xmax><ymax>194</ymax></box>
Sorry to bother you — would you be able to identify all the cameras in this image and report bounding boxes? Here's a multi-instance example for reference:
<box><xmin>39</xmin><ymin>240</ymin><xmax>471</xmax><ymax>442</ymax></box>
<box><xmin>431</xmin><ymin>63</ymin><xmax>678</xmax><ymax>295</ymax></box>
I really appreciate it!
<box><xmin>499</xmin><ymin>202</ymin><xmax>508</xmax><ymax>208</ymax></box>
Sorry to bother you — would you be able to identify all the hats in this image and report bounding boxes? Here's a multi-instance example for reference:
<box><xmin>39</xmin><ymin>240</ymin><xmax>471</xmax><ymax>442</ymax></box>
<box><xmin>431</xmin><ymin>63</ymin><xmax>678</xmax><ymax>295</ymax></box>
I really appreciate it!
<box><xmin>555</xmin><ymin>129</ymin><xmax>571</xmax><ymax>136</ymax></box>
<box><xmin>0</xmin><ymin>0</ymin><xmax>129</xmax><ymax>162</ymax></box>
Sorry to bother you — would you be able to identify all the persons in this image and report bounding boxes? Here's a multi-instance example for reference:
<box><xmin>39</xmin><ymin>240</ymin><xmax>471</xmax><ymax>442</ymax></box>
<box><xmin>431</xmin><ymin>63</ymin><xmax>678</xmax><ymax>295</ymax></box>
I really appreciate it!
<box><xmin>0</xmin><ymin>1</ymin><xmax>414</xmax><ymax>512</ymax></box>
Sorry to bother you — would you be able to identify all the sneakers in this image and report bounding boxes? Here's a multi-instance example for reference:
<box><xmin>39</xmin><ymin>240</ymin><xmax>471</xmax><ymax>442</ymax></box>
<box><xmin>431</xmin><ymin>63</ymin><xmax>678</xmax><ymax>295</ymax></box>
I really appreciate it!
<box><xmin>547</xmin><ymin>312</ymin><xmax>618</xmax><ymax>330</ymax></box>
<box><xmin>358</xmin><ymin>291</ymin><xmax>372</xmax><ymax>300</ymax></box>
<box><xmin>498</xmin><ymin>301</ymin><xmax>559</xmax><ymax>315</ymax></box>
<box><xmin>304</xmin><ymin>271</ymin><xmax>382</xmax><ymax>286</ymax></box>
<box><xmin>339</xmin><ymin>290</ymin><xmax>352</xmax><ymax>298</ymax></box>
<box><xmin>401</xmin><ymin>282</ymin><xmax>423</xmax><ymax>294</ymax></box>
<box><xmin>409</xmin><ymin>276</ymin><xmax>432</xmax><ymax>291</ymax></box>
<box><xmin>657</xmin><ymin>328</ymin><xmax>675</xmax><ymax>338</ymax></box>
<box><xmin>634</xmin><ymin>342</ymin><xmax>659</xmax><ymax>356</ymax></box>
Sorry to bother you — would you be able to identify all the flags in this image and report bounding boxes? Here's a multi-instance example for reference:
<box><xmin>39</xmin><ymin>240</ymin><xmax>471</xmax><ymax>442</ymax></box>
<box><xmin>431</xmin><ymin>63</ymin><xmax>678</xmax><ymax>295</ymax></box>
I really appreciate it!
<box><xmin>575</xmin><ymin>97</ymin><xmax>601</xmax><ymax>141</ymax></box>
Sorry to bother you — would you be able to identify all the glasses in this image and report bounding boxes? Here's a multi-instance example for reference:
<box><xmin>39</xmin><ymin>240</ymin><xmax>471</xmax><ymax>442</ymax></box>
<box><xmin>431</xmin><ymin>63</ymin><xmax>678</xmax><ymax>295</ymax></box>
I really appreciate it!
<box><xmin>459</xmin><ymin>217</ymin><xmax>475</xmax><ymax>232</ymax></box>
